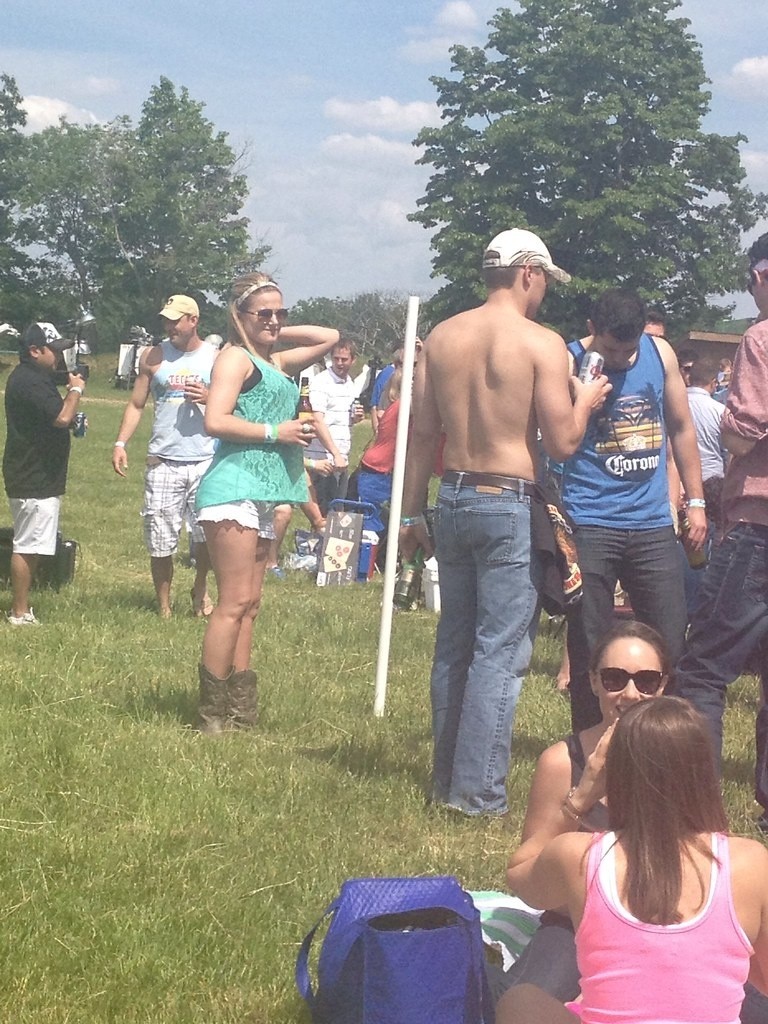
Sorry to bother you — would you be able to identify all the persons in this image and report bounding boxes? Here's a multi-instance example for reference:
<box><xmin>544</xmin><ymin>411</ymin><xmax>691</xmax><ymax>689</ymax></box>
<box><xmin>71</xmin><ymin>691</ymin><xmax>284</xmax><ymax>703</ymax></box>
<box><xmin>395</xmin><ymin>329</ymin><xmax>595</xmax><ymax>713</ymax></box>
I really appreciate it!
<box><xmin>671</xmin><ymin>233</ymin><xmax>768</xmax><ymax>830</ymax></box>
<box><xmin>2</xmin><ymin>322</ymin><xmax>87</xmax><ymax>627</ymax></box>
<box><xmin>195</xmin><ymin>270</ymin><xmax>339</xmax><ymax>732</ymax></box>
<box><xmin>494</xmin><ymin>694</ymin><xmax>768</xmax><ymax>1024</ymax></box>
<box><xmin>111</xmin><ymin>292</ymin><xmax>445</xmax><ymax>618</ymax></box>
<box><xmin>399</xmin><ymin>227</ymin><xmax>614</xmax><ymax>822</ymax></box>
<box><xmin>489</xmin><ymin>620</ymin><xmax>768</xmax><ymax>1024</ymax></box>
<box><xmin>540</xmin><ymin>282</ymin><xmax>708</xmax><ymax>733</ymax></box>
<box><xmin>540</xmin><ymin>307</ymin><xmax>735</xmax><ymax>691</ymax></box>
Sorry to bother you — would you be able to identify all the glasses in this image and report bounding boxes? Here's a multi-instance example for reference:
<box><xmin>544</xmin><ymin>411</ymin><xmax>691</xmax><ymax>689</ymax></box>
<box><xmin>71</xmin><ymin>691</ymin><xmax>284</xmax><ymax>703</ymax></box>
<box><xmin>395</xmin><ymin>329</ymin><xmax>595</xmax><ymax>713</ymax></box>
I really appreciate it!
<box><xmin>594</xmin><ymin>667</ymin><xmax>666</xmax><ymax>694</ymax></box>
<box><xmin>244</xmin><ymin>308</ymin><xmax>289</xmax><ymax>320</ymax></box>
<box><xmin>747</xmin><ymin>278</ymin><xmax>757</xmax><ymax>293</ymax></box>
<box><xmin>682</xmin><ymin>365</ymin><xmax>691</xmax><ymax>372</ymax></box>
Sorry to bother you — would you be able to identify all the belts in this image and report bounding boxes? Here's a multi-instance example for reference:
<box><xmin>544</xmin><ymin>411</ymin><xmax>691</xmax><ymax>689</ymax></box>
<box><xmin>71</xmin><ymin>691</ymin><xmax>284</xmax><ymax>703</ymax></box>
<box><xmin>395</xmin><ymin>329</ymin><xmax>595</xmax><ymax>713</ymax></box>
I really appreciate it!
<box><xmin>442</xmin><ymin>471</ymin><xmax>535</xmax><ymax>496</ymax></box>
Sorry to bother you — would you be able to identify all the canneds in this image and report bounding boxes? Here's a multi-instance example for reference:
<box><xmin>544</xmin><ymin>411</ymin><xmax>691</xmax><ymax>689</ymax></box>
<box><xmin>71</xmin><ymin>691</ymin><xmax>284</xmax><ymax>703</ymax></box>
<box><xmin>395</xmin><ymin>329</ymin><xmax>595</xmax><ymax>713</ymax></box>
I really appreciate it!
<box><xmin>578</xmin><ymin>351</ymin><xmax>604</xmax><ymax>384</ymax></box>
<box><xmin>183</xmin><ymin>377</ymin><xmax>196</xmax><ymax>399</ymax></box>
<box><xmin>73</xmin><ymin>412</ymin><xmax>86</xmax><ymax>437</ymax></box>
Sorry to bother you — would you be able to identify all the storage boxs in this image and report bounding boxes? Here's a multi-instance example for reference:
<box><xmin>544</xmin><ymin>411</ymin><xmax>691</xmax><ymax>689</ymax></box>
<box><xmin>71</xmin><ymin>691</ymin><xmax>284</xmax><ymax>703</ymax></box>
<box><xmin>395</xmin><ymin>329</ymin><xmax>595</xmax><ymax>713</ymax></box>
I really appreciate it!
<box><xmin>421</xmin><ymin>568</ymin><xmax>441</xmax><ymax>612</ymax></box>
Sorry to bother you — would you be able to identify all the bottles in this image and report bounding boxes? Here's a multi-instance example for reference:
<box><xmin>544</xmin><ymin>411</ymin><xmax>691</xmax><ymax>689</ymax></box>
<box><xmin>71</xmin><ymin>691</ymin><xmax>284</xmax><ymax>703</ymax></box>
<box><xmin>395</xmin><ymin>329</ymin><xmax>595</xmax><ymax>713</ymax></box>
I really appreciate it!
<box><xmin>392</xmin><ymin>546</ymin><xmax>425</xmax><ymax>607</ymax></box>
<box><xmin>679</xmin><ymin>512</ymin><xmax>708</xmax><ymax>570</ymax></box>
<box><xmin>295</xmin><ymin>377</ymin><xmax>312</xmax><ymax>444</ymax></box>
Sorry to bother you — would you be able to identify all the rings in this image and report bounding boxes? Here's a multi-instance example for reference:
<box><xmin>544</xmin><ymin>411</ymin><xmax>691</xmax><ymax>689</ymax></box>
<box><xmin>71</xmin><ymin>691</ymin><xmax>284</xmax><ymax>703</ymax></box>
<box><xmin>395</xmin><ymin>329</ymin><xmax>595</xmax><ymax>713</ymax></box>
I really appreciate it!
<box><xmin>303</xmin><ymin>423</ymin><xmax>311</xmax><ymax>432</ymax></box>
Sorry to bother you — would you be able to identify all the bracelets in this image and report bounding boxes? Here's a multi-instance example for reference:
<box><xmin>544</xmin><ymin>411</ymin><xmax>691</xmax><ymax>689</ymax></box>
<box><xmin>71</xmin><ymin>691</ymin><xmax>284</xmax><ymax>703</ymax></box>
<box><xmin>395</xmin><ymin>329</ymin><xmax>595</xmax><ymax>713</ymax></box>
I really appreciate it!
<box><xmin>401</xmin><ymin>516</ymin><xmax>425</xmax><ymax>526</ymax></box>
<box><xmin>115</xmin><ymin>441</ymin><xmax>125</xmax><ymax>446</ymax></box>
<box><xmin>265</xmin><ymin>424</ymin><xmax>278</xmax><ymax>444</ymax></box>
<box><xmin>687</xmin><ymin>499</ymin><xmax>706</xmax><ymax>508</ymax></box>
<box><xmin>69</xmin><ymin>386</ymin><xmax>83</xmax><ymax>395</ymax></box>
<box><xmin>560</xmin><ymin>786</ymin><xmax>587</xmax><ymax>822</ymax></box>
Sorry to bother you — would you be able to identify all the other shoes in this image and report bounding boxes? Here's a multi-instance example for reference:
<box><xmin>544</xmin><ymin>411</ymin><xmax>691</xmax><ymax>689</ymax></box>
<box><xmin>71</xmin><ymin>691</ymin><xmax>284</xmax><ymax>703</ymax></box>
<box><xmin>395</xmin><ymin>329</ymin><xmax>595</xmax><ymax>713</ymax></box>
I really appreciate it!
<box><xmin>8</xmin><ymin>607</ymin><xmax>38</xmax><ymax>625</ymax></box>
<box><xmin>266</xmin><ymin>566</ymin><xmax>286</xmax><ymax>579</ymax></box>
<box><xmin>191</xmin><ymin>588</ymin><xmax>213</xmax><ymax>617</ymax></box>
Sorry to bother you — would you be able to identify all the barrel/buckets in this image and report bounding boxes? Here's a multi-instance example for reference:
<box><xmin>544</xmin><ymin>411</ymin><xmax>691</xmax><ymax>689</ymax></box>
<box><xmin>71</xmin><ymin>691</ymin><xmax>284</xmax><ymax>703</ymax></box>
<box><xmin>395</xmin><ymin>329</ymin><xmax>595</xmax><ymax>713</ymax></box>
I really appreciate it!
<box><xmin>421</xmin><ymin>557</ymin><xmax>441</xmax><ymax>612</ymax></box>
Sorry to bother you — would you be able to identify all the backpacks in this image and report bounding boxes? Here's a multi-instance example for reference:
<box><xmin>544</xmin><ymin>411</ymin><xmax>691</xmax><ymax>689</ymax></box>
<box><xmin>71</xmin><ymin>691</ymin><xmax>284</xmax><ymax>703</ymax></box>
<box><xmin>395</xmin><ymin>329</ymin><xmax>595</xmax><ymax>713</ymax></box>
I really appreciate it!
<box><xmin>295</xmin><ymin>877</ymin><xmax>494</xmax><ymax>1024</ymax></box>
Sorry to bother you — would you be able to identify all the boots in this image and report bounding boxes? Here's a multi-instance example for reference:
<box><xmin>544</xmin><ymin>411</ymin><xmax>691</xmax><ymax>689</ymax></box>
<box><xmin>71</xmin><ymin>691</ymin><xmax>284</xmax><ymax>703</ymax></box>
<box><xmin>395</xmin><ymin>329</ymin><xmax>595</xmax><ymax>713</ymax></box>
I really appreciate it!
<box><xmin>195</xmin><ymin>663</ymin><xmax>234</xmax><ymax>737</ymax></box>
<box><xmin>227</xmin><ymin>669</ymin><xmax>258</xmax><ymax>730</ymax></box>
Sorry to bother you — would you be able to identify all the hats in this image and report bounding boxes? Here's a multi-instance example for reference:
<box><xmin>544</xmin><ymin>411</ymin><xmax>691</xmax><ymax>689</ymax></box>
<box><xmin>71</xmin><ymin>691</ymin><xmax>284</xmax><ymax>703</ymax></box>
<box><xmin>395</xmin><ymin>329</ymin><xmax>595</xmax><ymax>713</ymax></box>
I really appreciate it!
<box><xmin>159</xmin><ymin>295</ymin><xmax>200</xmax><ymax>320</ymax></box>
<box><xmin>27</xmin><ymin>323</ymin><xmax>76</xmax><ymax>350</ymax></box>
<box><xmin>482</xmin><ymin>228</ymin><xmax>572</xmax><ymax>284</ymax></box>
